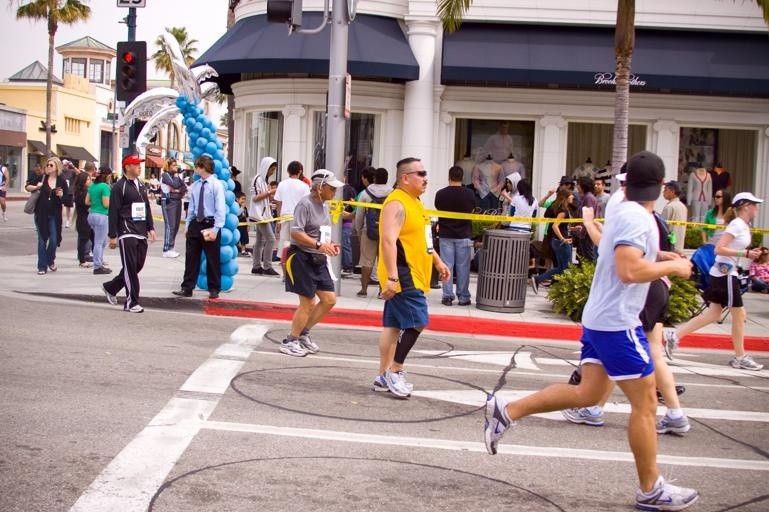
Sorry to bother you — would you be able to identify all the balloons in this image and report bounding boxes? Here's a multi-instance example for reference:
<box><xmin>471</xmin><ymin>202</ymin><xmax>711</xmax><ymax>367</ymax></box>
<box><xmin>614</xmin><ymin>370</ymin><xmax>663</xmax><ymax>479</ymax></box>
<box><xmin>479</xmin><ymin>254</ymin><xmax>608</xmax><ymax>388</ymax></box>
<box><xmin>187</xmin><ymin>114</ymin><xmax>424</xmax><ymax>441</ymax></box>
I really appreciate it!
<box><xmin>175</xmin><ymin>91</ymin><xmax>242</xmax><ymax>293</ymax></box>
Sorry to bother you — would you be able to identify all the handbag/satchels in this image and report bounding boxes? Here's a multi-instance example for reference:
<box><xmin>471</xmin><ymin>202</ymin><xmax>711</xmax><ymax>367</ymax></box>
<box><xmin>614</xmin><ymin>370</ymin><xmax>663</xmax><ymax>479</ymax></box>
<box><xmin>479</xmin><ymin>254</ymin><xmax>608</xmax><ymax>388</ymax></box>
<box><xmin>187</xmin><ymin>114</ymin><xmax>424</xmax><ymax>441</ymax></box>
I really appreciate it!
<box><xmin>24</xmin><ymin>190</ymin><xmax>40</xmax><ymax>214</ymax></box>
<box><xmin>161</xmin><ymin>183</ymin><xmax>172</xmax><ymax>193</ymax></box>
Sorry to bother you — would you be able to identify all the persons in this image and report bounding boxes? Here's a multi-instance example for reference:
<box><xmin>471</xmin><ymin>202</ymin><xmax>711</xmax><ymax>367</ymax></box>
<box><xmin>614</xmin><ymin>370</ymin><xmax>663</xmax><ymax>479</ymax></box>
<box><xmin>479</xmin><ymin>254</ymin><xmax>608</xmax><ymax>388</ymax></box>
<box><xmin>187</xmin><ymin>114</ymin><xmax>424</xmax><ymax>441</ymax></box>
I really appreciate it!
<box><xmin>484</xmin><ymin>149</ymin><xmax>699</xmax><ymax>511</ymax></box>
<box><xmin>498</xmin><ymin>170</ymin><xmax>522</xmax><ymax>229</ymax></box>
<box><xmin>510</xmin><ymin>178</ymin><xmax>539</xmax><ymax>231</ymax></box>
<box><xmin>341</xmin><ymin>164</ymin><xmax>392</xmax><ymax>300</ymax></box>
<box><xmin>471</xmin><ymin>153</ymin><xmax>507</xmax><ymax>212</ymax></box>
<box><xmin>178</xmin><ymin>155</ymin><xmax>226</xmax><ymax>298</ymax></box>
<box><xmin>100</xmin><ymin>155</ymin><xmax>156</xmax><ymax>312</ymax></box>
<box><xmin>501</xmin><ymin>152</ymin><xmax>529</xmax><ymax>183</ymax></box>
<box><xmin>230</xmin><ymin>157</ymin><xmax>310</xmax><ymax>279</ymax></box>
<box><xmin>435</xmin><ymin>164</ymin><xmax>478</xmax><ymax>306</ymax></box>
<box><xmin>149</xmin><ymin>158</ymin><xmax>192</xmax><ymax>259</ymax></box>
<box><xmin>25</xmin><ymin>155</ymin><xmax>120</xmax><ymax>275</ymax></box>
<box><xmin>0</xmin><ymin>157</ymin><xmax>10</xmax><ymax>224</ymax></box>
<box><xmin>534</xmin><ymin>158</ymin><xmax>768</xmax><ymax>434</ymax></box>
<box><xmin>279</xmin><ymin>169</ymin><xmax>344</xmax><ymax>357</ymax></box>
<box><xmin>458</xmin><ymin>153</ymin><xmax>477</xmax><ymax>199</ymax></box>
<box><xmin>371</xmin><ymin>157</ymin><xmax>451</xmax><ymax>400</ymax></box>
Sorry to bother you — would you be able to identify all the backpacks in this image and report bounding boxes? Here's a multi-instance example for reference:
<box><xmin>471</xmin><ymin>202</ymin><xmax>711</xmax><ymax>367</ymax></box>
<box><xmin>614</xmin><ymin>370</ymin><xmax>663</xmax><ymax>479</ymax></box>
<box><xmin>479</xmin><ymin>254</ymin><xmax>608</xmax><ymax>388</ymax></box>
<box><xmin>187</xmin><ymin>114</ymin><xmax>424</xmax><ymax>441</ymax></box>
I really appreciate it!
<box><xmin>364</xmin><ymin>188</ymin><xmax>387</xmax><ymax>240</ymax></box>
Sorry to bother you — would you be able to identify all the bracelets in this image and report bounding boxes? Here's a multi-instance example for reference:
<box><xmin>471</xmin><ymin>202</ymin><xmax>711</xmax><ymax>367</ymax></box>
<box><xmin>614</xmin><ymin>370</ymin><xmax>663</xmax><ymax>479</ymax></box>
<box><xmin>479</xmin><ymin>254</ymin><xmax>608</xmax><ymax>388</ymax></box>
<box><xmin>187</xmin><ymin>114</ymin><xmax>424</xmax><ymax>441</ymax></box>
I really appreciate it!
<box><xmin>388</xmin><ymin>277</ymin><xmax>399</xmax><ymax>282</ymax></box>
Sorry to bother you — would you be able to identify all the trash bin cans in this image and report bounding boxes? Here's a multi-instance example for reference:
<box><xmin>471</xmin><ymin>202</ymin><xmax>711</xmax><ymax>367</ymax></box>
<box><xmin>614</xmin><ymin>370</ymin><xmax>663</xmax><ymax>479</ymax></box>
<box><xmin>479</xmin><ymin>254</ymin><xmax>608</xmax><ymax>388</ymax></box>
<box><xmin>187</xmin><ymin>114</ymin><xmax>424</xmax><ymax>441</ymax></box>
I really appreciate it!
<box><xmin>475</xmin><ymin>228</ymin><xmax>531</xmax><ymax>313</ymax></box>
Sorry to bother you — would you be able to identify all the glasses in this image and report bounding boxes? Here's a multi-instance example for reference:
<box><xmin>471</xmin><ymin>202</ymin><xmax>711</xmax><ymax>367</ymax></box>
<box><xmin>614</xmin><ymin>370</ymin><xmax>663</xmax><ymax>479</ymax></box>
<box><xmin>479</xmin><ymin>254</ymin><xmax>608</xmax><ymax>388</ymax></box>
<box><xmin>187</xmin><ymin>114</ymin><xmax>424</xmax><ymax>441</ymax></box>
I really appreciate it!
<box><xmin>715</xmin><ymin>194</ymin><xmax>722</xmax><ymax>199</ymax></box>
<box><xmin>560</xmin><ymin>183</ymin><xmax>569</xmax><ymax>186</ymax></box>
<box><xmin>46</xmin><ymin>164</ymin><xmax>53</xmax><ymax>168</ymax></box>
<box><xmin>406</xmin><ymin>170</ymin><xmax>427</xmax><ymax>177</ymax></box>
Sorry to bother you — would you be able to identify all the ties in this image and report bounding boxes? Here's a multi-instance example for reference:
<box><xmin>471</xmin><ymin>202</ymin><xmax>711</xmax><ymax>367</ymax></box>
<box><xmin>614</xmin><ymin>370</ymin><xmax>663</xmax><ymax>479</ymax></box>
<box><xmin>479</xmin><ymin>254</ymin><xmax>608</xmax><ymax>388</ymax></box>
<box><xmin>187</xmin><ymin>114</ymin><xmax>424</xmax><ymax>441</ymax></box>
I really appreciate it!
<box><xmin>198</xmin><ymin>180</ymin><xmax>208</xmax><ymax>221</ymax></box>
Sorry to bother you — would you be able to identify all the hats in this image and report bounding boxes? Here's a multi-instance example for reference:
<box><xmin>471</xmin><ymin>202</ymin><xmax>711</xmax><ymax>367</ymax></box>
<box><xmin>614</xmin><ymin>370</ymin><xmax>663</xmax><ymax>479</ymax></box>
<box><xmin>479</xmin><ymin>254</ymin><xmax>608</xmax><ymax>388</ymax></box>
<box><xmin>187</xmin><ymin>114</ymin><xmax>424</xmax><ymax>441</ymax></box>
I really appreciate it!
<box><xmin>558</xmin><ymin>176</ymin><xmax>575</xmax><ymax>186</ymax></box>
<box><xmin>122</xmin><ymin>156</ymin><xmax>146</xmax><ymax>168</ymax></box>
<box><xmin>663</xmin><ymin>180</ymin><xmax>682</xmax><ymax>193</ymax></box>
<box><xmin>625</xmin><ymin>151</ymin><xmax>665</xmax><ymax>201</ymax></box>
<box><xmin>731</xmin><ymin>193</ymin><xmax>765</xmax><ymax>207</ymax></box>
<box><xmin>98</xmin><ymin>166</ymin><xmax>113</xmax><ymax>176</ymax></box>
<box><xmin>312</xmin><ymin>169</ymin><xmax>346</xmax><ymax>188</ymax></box>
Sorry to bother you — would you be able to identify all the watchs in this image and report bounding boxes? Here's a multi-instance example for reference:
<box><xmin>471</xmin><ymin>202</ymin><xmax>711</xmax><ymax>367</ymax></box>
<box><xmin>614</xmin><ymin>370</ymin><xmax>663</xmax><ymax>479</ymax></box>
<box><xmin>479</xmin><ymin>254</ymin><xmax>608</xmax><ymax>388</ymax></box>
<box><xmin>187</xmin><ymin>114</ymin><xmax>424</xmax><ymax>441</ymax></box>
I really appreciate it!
<box><xmin>315</xmin><ymin>240</ymin><xmax>322</xmax><ymax>249</ymax></box>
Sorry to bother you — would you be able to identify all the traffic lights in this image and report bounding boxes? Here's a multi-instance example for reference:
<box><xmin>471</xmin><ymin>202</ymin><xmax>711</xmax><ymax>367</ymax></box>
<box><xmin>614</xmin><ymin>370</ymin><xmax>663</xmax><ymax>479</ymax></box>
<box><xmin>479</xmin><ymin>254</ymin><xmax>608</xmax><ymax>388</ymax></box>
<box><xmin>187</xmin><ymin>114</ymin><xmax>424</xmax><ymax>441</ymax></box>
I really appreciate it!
<box><xmin>115</xmin><ymin>40</ymin><xmax>148</xmax><ymax>101</ymax></box>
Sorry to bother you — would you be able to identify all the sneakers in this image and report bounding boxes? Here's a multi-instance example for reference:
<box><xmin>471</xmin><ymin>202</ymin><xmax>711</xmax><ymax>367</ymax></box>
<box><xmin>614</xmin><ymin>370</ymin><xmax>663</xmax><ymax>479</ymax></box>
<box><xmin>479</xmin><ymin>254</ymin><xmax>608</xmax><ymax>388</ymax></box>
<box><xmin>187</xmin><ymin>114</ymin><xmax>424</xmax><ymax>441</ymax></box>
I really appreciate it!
<box><xmin>252</xmin><ymin>266</ymin><xmax>265</xmax><ymax>276</ymax></box>
<box><xmin>298</xmin><ymin>335</ymin><xmax>320</xmax><ymax>354</ymax></box>
<box><xmin>357</xmin><ymin>291</ymin><xmax>367</xmax><ymax>297</ymax></box>
<box><xmin>372</xmin><ymin>374</ymin><xmax>413</xmax><ymax>398</ymax></box>
<box><xmin>634</xmin><ymin>476</ymin><xmax>700</xmax><ymax>511</ymax></box>
<box><xmin>656</xmin><ymin>385</ymin><xmax>685</xmax><ymax>404</ymax></box>
<box><xmin>264</xmin><ymin>268</ymin><xmax>280</xmax><ymax>278</ymax></box>
<box><xmin>655</xmin><ymin>415</ymin><xmax>691</xmax><ymax>437</ymax></box>
<box><xmin>38</xmin><ymin>262</ymin><xmax>57</xmax><ymax>275</ymax></box>
<box><xmin>164</xmin><ymin>250</ymin><xmax>180</xmax><ymax>257</ymax></box>
<box><xmin>662</xmin><ymin>327</ymin><xmax>678</xmax><ymax>360</ymax></box>
<box><xmin>730</xmin><ymin>353</ymin><xmax>764</xmax><ymax>372</ymax></box>
<box><xmin>102</xmin><ymin>284</ymin><xmax>118</xmax><ymax>305</ymax></box>
<box><xmin>563</xmin><ymin>372</ymin><xmax>605</xmax><ymax>426</ymax></box>
<box><xmin>239</xmin><ymin>251</ymin><xmax>251</xmax><ymax>257</ymax></box>
<box><xmin>485</xmin><ymin>393</ymin><xmax>514</xmax><ymax>456</ymax></box>
<box><xmin>531</xmin><ymin>275</ymin><xmax>542</xmax><ymax>293</ymax></box>
<box><xmin>81</xmin><ymin>258</ymin><xmax>110</xmax><ymax>274</ymax></box>
<box><xmin>280</xmin><ymin>339</ymin><xmax>308</xmax><ymax>357</ymax></box>
<box><xmin>65</xmin><ymin>218</ymin><xmax>75</xmax><ymax>229</ymax></box>
<box><xmin>124</xmin><ymin>304</ymin><xmax>144</xmax><ymax>313</ymax></box>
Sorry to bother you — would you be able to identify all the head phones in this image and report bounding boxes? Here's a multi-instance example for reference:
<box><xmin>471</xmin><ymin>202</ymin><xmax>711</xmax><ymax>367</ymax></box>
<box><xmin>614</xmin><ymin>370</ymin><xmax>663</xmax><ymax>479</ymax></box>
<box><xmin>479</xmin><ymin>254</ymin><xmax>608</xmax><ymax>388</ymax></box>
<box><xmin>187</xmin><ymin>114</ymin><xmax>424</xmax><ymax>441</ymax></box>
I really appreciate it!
<box><xmin>318</xmin><ymin>170</ymin><xmax>329</xmax><ymax>194</ymax></box>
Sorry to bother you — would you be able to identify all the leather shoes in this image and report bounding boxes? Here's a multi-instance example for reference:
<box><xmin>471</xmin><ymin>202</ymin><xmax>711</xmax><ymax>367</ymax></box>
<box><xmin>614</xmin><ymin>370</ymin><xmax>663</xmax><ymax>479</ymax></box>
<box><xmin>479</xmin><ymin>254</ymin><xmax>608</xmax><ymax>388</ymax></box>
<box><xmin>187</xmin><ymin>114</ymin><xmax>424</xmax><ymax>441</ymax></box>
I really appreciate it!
<box><xmin>172</xmin><ymin>289</ymin><xmax>193</xmax><ymax>296</ymax></box>
<box><xmin>209</xmin><ymin>289</ymin><xmax>219</xmax><ymax>298</ymax></box>
<box><xmin>442</xmin><ymin>298</ymin><xmax>452</xmax><ymax>306</ymax></box>
<box><xmin>458</xmin><ymin>299</ymin><xmax>471</xmax><ymax>306</ymax></box>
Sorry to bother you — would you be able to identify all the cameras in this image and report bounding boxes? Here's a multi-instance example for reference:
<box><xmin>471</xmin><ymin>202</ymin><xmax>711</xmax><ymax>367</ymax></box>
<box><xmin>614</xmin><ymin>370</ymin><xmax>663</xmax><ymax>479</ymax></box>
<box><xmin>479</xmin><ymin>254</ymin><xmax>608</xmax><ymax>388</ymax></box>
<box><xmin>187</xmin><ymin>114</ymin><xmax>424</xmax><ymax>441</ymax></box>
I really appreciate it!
<box><xmin>178</xmin><ymin>168</ymin><xmax>185</xmax><ymax>173</ymax></box>
